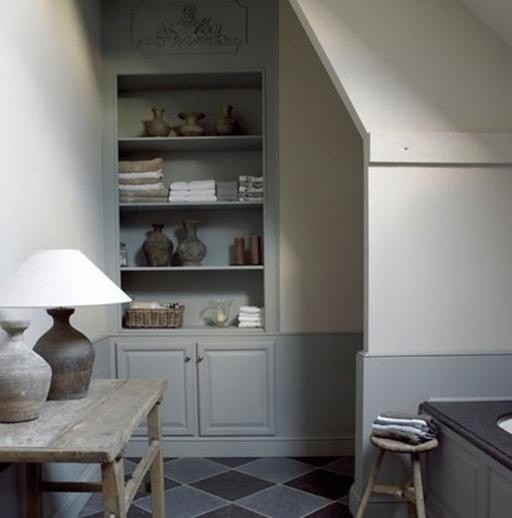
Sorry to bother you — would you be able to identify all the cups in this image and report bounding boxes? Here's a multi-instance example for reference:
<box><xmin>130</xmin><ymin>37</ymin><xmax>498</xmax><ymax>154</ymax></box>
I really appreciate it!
<box><xmin>206</xmin><ymin>294</ymin><xmax>235</xmax><ymax>328</ymax></box>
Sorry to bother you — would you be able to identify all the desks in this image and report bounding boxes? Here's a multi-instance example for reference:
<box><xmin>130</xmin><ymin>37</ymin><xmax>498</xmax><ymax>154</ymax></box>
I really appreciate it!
<box><xmin>0</xmin><ymin>376</ymin><xmax>168</xmax><ymax>516</ymax></box>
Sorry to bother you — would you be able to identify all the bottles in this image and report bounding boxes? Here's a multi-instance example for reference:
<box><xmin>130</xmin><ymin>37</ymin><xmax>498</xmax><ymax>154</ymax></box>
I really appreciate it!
<box><xmin>142</xmin><ymin>222</ymin><xmax>175</xmax><ymax>267</ymax></box>
<box><xmin>120</xmin><ymin>240</ymin><xmax>129</xmax><ymax>268</ymax></box>
<box><xmin>144</xmin><ymin>104</ymin><xmax>242</xmax><ymax>138</ymax></box>
<box><xmin>176</xmin><ymin>218</ymin><xmax>208</xmax><ymax>268</ymax></box>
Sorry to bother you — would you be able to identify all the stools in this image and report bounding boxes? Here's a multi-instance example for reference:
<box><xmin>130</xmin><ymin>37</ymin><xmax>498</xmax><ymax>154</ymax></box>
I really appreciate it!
<box><xmin>355</xmin><ymin>433</ymin><xmax>438</xmax><ymax>518</ymax></box>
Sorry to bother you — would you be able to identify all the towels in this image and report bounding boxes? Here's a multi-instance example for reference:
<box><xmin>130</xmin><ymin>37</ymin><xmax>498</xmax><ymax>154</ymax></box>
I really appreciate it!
<box><xmin>371</xmin><ymin>411</ymin><xmax>440</xmax><ymax>446</ymax></box>
<box><xmin>118</xmin><ymin>157</ymin><xmax>263</xmax><ymax>202</ymax></box>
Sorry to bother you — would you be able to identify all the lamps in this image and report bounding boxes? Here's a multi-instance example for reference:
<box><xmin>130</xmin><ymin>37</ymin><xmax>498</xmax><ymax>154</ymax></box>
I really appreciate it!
<box><xmin>1</xmin><ymin>250</ymin><xmax>131</xmax><ymax>401</ymax></box>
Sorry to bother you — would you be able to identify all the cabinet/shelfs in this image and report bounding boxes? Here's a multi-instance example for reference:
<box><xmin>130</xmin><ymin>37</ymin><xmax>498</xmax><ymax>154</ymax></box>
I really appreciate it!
<box><xmin>100</xmin><ymin>0</ymin><xmax>278</xmax><ymax>457</ymax></box>
<box><xmin>419</xmin><ymin>410</ymin><xmax>512</xmax><ymax>517</ymax></box>
